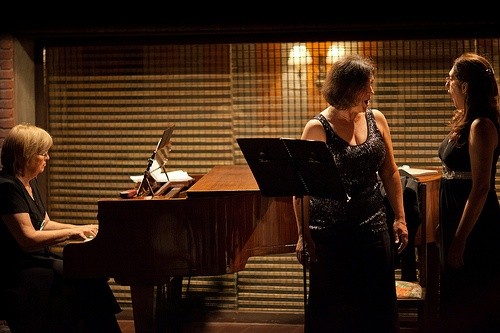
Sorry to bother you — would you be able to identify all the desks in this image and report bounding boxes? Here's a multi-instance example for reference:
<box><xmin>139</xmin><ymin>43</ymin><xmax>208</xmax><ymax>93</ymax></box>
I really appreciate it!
<box><xmin>413</xmin><ymin>174</ymin><xmax>441</xmax><ymax>319</ymax></box>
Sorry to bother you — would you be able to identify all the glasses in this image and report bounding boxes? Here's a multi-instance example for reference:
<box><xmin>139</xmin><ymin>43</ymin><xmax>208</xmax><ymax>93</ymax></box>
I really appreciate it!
<box><xmin>33</xmin><ymin>150</ymin><xmax>49</xmax><ymax>157</ymax></box>
<box><xmin>446</xmin><ymin>77</ymin><xmax>464</xmax><ymax>82</ymax></box>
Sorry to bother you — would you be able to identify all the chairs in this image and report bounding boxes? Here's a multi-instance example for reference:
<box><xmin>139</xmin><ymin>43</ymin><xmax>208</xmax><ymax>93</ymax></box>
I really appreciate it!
<box><xmin>377</xmin><ymin>169</ymin><xmax>426</xmax><ymax>333</ymax></box>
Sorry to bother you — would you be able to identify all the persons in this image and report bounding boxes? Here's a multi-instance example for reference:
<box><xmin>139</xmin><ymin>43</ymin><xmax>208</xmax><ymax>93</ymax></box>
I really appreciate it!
<box><xmin>1</xmin><ymin>121</ymin><xmax>123</xmax><ymax>333</ymax></box>
<box><xmin>433</xmin><ymin>52</ymin><xmax>500</xmax><ymax>333</ymax></box>
<box><xmin>292</xmin><ymin>54</ymin><xmax>409</xmax><ymax>333</ymax></box>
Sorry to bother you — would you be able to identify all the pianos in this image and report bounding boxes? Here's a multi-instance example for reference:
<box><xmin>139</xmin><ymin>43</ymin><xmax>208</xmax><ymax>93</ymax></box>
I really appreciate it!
<box><xmin>63</xmin><ymin>165</ymin><xmax>441</xmax><ymax>333</ymax></box>
